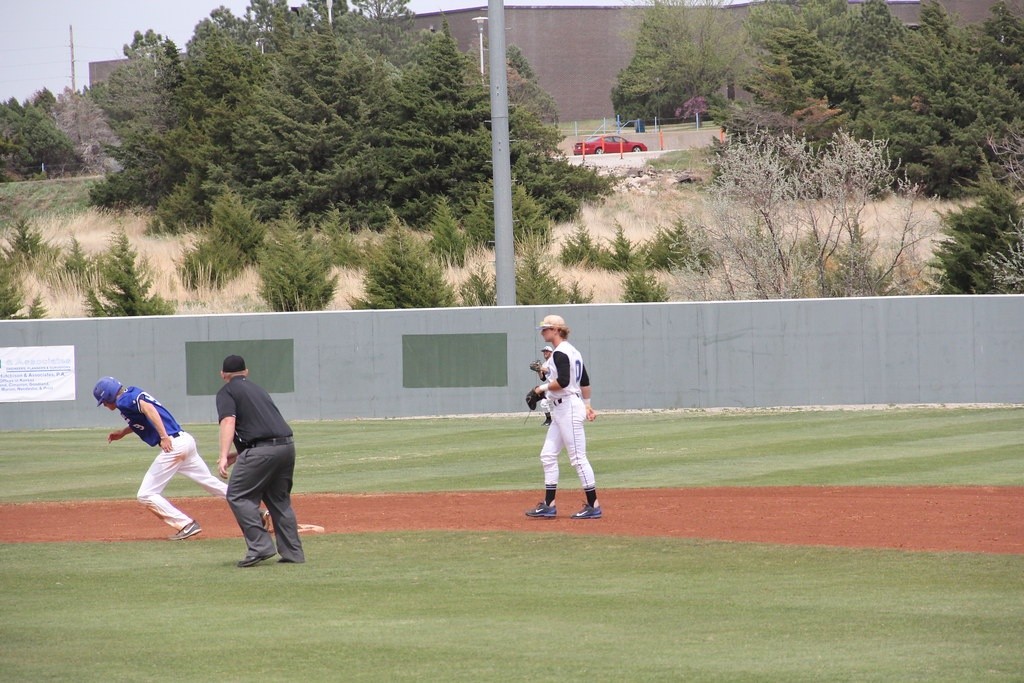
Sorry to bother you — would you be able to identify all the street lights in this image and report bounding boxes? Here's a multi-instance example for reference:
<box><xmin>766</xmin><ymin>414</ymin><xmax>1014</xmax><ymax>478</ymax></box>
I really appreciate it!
<box><xmin>472</xmin><ymin>16</ymin><xmax>488</xmax><ymax>85</ymax></box>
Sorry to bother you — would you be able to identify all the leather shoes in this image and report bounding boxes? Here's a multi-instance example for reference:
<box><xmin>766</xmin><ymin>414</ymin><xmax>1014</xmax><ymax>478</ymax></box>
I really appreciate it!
<box><xmin>278</xmin><ymin>558</ymin><xmax>293</xmax><ymax>563</ymax></box>
<box><xmin>238</xmin><ymin>554</ymin><xmax>275</xmax><ymax>567</ymax></box>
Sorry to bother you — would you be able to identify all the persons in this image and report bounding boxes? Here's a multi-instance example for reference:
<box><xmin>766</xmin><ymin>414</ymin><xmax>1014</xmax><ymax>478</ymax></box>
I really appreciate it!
<box><xmin>534</xmin><ymin>346</ymin><xmax>562</xmax><ymax>426</ymax></box>
<box><xmin>93</xmin><ymin>376</ymin><xmax>271</xmax><ymax>540</ymax></box>
<box><xmin>525</xmin><ymin>315</ymin><xmax>602</xmax><ymax>519</ymax></box>
<box><xmin>216</xmin><ymin>355</ymin><xmax>306</xmax><ymax>567</ymax></box>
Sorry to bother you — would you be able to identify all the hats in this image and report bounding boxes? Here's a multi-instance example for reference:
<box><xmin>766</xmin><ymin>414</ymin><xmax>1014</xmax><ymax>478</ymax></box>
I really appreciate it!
<box><xmin>535</xmin><ymin>315</ymin><xmax>566</xmax><ymax>330</ymax></box>
<box><xmin>541</xmin><ymin>346</ymin><xmax>553</xmax><ymax>352</ymax></box>
<box><xmin>223</xmin><ymin>355</ymin><xmax>246</xmax><ymax>372</ymax></box>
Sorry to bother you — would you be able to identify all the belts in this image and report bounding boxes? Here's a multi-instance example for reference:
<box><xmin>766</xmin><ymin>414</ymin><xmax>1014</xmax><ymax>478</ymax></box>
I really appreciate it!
<box><xmin>251</xmin><ymin>437</ymin><xmax>293</xmax><ymax>448</ymax></box>
<box><xmin>553</xmin><ymin>394</ymin><xmax>579</xmax><ymax>405</ymax></box>
<box><xmin>173</xmin><ymin>429</ymin><xmax>183</xmax><ymax>438</ymax></box>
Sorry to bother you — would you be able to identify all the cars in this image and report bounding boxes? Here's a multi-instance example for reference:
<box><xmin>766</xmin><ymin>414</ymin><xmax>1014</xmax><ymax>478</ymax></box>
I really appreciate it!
<box><xmin>574</xmin><ymin>135</ymin><xmax>648</xmax><ymax>155</ymax></box>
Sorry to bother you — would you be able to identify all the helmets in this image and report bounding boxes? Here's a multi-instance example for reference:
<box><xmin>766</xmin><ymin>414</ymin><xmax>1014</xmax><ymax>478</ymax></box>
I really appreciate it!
<box><xmin>93</xmin><ymin>376</ymin><xmax>122</xmax><ymax>407</ymax></box>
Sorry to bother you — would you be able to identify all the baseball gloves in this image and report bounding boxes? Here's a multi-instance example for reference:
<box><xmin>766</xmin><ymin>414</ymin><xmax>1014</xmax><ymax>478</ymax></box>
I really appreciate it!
<box><xmin>529</xmin><ymin>359</ymin><xmax>546</xmax><ymax>382</ymax></box>
<box><xmin>524</xmin><ymin>385</ymin><xmax>547</xmax><ymax>425</ymax></box>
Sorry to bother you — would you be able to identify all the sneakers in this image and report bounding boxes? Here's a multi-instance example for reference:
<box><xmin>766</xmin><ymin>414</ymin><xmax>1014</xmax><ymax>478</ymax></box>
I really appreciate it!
<box><xmin>169</xmin><ymin>520</ymin><xmax>202</xmax><ymax>540</ymax></box>
<box><xmin>525</xmin><ymin>502</ymin><xmax>556</xmax><ymax>517</ymax></box>
<box><xmin>571</xmin><ymin>505</ymin><xmax>601</xmax><ymax>519</ymax></box>
<box><xmin>258</xmin><ymin>508</ymin><xmax>270</xmax><ymax>530</ymax></box>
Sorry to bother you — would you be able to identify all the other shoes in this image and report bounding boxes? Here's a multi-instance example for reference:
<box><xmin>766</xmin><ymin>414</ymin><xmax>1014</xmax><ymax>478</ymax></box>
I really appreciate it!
<box><xmin>542</xmin><ymin>419</ymin><xmax>552</xmax><ymax>425</ymax></box>
